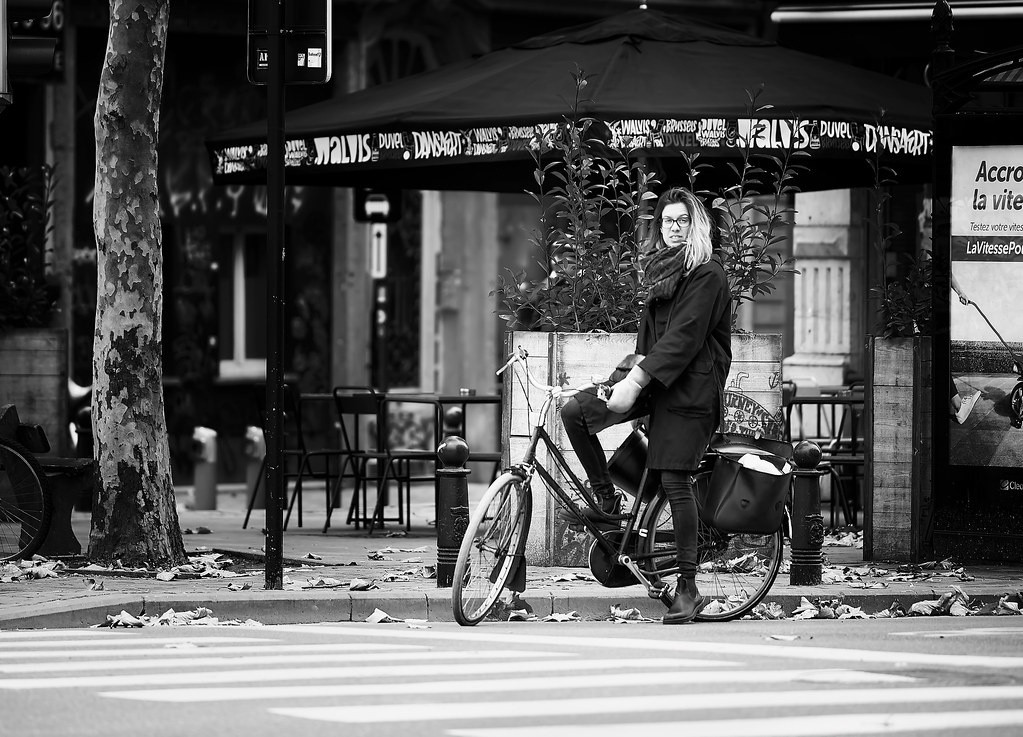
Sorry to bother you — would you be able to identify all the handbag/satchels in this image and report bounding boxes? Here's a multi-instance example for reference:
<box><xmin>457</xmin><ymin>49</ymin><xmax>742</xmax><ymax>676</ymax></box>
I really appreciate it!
<box><xmin>701</xmin><ymin>452</ymin><xmax>793</xmax><ymax>535</ymax></box>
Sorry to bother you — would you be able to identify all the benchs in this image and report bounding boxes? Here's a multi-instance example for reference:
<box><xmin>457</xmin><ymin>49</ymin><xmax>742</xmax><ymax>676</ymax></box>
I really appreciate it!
<box><xmin>0</xmin><ymin>402</ymin><xmax>95</xmax><ymax>557</ymax></box>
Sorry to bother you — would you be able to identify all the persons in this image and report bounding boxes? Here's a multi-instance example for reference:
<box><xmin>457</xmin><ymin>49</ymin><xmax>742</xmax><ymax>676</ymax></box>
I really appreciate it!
<box><xmin>951</xmin><ymin>273</ymin><xmax>980</xmax><ymax>426</ymax></box>
<box><xmin>560</xmin><ymin>189</ymin><xmax>734</xmax><ymax>625</ymax></box>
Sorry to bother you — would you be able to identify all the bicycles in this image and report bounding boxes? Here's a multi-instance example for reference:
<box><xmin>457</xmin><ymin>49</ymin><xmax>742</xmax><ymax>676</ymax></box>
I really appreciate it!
<box><xmin>452</xmin><ymin>344</ymin><xmax>799</xmax><ymax>628</ymax></box>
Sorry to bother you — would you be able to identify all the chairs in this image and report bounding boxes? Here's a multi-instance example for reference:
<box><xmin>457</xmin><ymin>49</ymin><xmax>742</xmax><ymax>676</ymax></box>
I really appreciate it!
<box><xmin>777</xmin><ymin>381</ymin><xmax>863</xmax><ymax>529</ymax></box>
<box><xmin>241</xmin><ymin>379</ymin><xmax>450</xmax><ymax>526</ymax></box>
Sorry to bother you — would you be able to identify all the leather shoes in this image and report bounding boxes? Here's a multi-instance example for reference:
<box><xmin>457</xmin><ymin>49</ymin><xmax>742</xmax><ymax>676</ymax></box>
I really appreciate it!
<box><xmin>663</xmin><ymin>577</ymin><xmax>704</xmax><ymax>624</ymax></box>
<box><xmin>558</xmin><ymin>496</ymin><xmax>622</xmax><ymax>531</ymax></box>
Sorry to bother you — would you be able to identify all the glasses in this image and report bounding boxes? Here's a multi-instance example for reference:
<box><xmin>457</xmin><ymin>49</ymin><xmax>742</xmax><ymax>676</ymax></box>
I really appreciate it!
<box><xmin>659</xmin><ymin>214</ymin><xmax>691</xmax><ymax>229</ymax></box>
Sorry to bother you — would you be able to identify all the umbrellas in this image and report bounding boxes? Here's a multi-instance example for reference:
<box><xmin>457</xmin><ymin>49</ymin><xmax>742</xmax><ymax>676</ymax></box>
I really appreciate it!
<box><xmin>207</xmin><ymin>0</ymin><xmax>935</xmax><ymax>333</ymax></box>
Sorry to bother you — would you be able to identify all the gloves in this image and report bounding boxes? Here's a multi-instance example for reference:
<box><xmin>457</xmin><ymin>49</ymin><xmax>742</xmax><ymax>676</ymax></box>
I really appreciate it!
<box><xmin>606</xmin><ymin>365</ymin><xmax>651</xmax><ymax>414</ymax></box>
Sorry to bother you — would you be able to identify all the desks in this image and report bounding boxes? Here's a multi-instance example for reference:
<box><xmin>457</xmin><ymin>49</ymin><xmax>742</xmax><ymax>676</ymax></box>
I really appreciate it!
<box><xmin>792</xmin><ymin>391</ymin><xmax>863</xmax><ymax>528</ymax></box>
<box><xmin>301</xmin><ymin>383</ymin><xmax>500</xmax><ymax>534</ymax></box>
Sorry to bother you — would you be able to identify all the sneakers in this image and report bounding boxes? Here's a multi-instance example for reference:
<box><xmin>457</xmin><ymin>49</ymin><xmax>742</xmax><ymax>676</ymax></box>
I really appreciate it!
<box><xmin>955</xmin><ymin>390</ymin><xmax>982</xmax><ymax>425</ymax></box>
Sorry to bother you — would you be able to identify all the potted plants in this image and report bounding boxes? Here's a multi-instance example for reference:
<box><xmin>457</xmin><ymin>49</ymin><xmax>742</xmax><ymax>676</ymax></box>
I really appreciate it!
<box><xmin>488</xmin><ymin>59</ymin><xmax>825</xmax><ymax>567</ymax></box>
